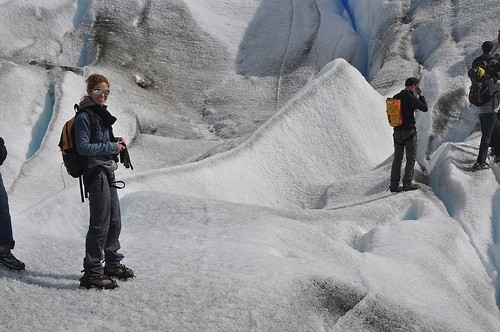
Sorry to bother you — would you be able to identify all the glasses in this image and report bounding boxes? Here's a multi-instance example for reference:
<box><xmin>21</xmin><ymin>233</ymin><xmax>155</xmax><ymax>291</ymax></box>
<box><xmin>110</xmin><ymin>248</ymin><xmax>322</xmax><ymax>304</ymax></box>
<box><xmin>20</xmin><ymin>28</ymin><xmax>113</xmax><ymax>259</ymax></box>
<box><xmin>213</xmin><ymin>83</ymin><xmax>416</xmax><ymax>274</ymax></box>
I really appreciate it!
<box><xmin>90</xmin><ymin>88</ymin><xmax>110</xmax><ymax>96</ymax></box>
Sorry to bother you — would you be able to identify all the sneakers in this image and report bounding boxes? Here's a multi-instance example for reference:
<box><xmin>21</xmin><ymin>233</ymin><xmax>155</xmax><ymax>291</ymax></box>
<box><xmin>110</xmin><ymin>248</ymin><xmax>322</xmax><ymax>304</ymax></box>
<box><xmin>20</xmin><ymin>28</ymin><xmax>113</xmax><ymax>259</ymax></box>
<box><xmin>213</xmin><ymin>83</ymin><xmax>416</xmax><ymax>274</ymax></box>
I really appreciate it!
<box><xmin>0</xmin><ymin>243</ymin><xmax>25</xmax><ymax>270</ymax></box>
<box><xmin>79</xmin><ymin>273</ymin><xmax>117</xmax><ymax>290</ymax></box>
<box><xmin>103</xmin><ymin>264</ymin><xmax>134</xmax><ymax>281</ymax></box>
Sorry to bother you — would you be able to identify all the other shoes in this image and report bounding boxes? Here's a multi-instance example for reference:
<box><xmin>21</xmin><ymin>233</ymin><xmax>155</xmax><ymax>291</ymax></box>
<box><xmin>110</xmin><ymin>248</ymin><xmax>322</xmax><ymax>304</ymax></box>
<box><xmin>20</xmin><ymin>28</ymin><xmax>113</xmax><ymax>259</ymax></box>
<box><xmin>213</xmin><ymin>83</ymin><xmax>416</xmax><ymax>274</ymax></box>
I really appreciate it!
<box><xmin>389</xmin><ymin>185</ymin><xmax>403</xmax><ymax>192</ymax></box>
<box><xmin>473</xmin><ymin>162</ymin><xmax>489</xmax><ymax>170</ymax></box>
<box><xmin>402</xmin><ymin>183</ymin><xmax>420</xmax><ymax>191</ymax></box>
<box><xmin>494</xmin><ymin>156</ymin><xmax>500</xmax><ymax>162</ymax></box>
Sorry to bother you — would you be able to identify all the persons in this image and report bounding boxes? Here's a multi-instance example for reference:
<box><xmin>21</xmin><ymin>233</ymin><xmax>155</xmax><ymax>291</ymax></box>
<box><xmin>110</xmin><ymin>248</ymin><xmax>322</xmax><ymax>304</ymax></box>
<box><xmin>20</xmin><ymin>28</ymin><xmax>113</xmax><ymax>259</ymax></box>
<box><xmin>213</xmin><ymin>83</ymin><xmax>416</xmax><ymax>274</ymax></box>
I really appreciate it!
<box><xmin>468</xmin><ymin>41</ymin><xmax>500</xmax><ymax>170</ymax></box>
<box><xmin>78</xmin><ymin>73</ymin><xmax>133</xmax><ymax>290</ymax></box>
<box><xmin>390</xmin><ymin>77</ymin><xmax>428</xmax><ymax>192</ymax></box>
<box><xmin>0</xmin><ymin>137</ymin><xmax>25</xmax><ymax>271</ymax></box>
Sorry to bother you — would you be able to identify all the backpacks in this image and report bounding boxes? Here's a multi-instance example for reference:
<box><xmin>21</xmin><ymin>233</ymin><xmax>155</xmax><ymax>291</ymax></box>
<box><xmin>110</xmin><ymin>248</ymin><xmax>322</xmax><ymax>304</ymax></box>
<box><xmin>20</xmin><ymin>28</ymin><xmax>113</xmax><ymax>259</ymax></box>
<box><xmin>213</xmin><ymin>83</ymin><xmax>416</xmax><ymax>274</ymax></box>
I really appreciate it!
<box><xmin>468</xmin><ymin>64</ymin><xmax>485</xmax><ymax>83</ymax></box>
<box><xmin>386</xmin><ymin>97</ymin><xmax>403</xmax><ymax>127</ymax></box>
<box><xmin>58</xmin><ymin>104</ymin><xmax>97</xmax><ymax>178</ymax></box>
<box><xmin>469</xmin><ymin>76</ymin><xmax>497</xmax><ymax>107</ymax></box>
